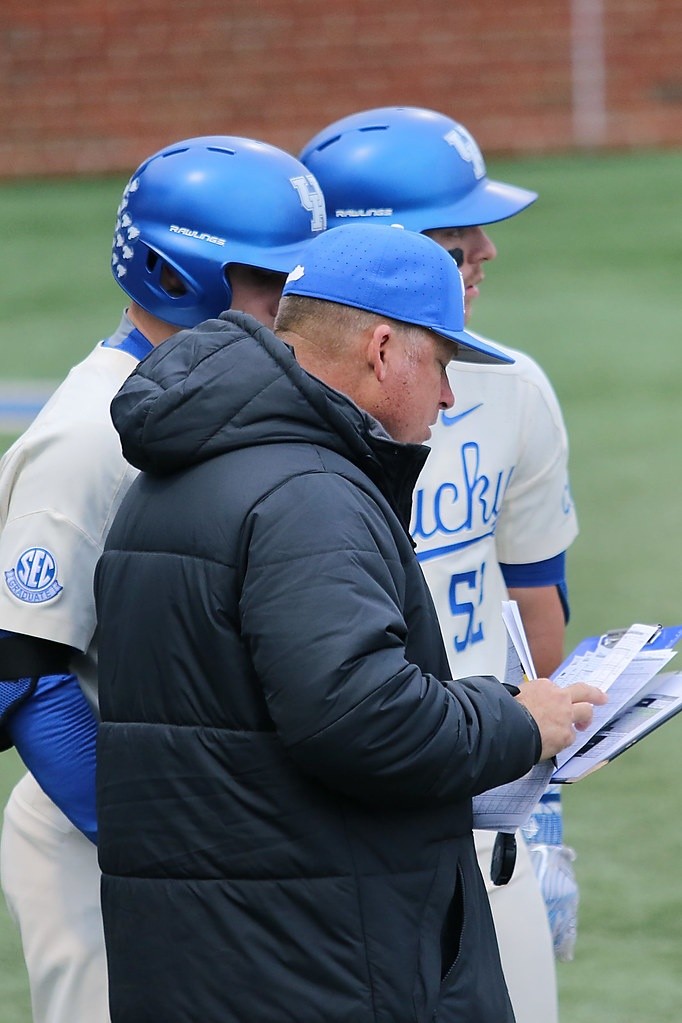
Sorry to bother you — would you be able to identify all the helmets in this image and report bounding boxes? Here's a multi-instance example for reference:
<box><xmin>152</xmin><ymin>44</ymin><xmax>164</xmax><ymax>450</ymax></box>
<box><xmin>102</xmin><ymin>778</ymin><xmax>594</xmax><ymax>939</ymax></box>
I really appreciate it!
<box><xmin>297</xmin><ymin>105</ymin><xmax>539</xmax><ymax>233</ymax></box>
<box><xmin>110</xmin><ymin>135</ymin><xmax>327</xmax><ymax>329</ymax></box>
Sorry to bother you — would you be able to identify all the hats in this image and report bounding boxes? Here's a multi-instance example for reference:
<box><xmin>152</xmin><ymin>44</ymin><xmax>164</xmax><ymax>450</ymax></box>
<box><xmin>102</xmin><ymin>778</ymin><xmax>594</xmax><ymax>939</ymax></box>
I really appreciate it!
<box><xmin>280</xmin><ymin>223</ymin><xmax>516</xmax><ymax>365</ymax></box>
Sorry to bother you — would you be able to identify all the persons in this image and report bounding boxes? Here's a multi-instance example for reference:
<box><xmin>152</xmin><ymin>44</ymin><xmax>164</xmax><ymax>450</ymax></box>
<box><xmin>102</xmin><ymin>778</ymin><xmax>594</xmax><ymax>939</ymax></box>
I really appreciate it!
<box><xmin>0</xmin><ymin>134</ymin><xmax>329</xmax><ymax>1023</ymax></box>
<box><xmin>298</xmin><ymin>105</ymin><xmax>581</xmax><ymax>1023</ymax></box>
<box><xmin>90</xmin><ymin>219</ymin><xmax>609</xmax><ymax>1022</ymax></box>
